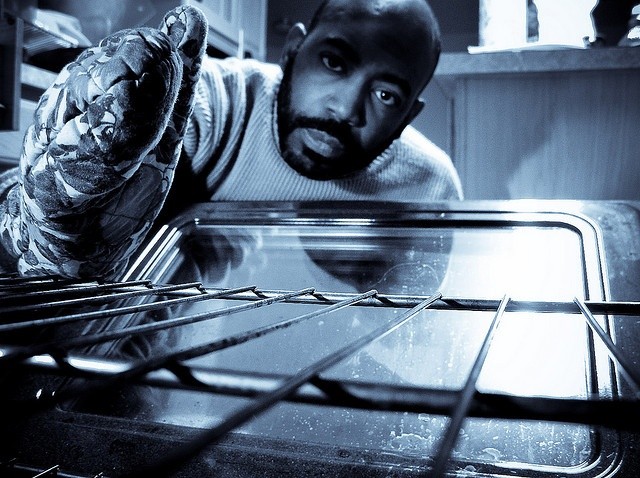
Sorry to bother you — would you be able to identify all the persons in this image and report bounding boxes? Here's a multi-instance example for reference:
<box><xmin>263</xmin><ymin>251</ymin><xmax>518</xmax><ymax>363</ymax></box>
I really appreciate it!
<box><xmin>0</xmin><ymin>1</ymin><xmax>464</xmax><ymax>288</ymax></box>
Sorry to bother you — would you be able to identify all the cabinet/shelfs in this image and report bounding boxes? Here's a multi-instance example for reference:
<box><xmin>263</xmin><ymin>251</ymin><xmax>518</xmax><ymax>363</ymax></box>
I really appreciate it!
<box><xmin>411</xmin><ymin>44</ymin><xmax>640</xmax><ymax>200</ymax></box>
<box><xmin>49</xmin><ymin>0</ymin><xmax>270</xmax><ymax>63</ymax></box>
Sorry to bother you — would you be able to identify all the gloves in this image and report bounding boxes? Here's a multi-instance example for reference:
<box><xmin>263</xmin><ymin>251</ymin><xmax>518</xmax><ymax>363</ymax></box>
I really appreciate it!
<box><xmin>1</xmin><ymin>4</ymin><xmax>208</xmax><ymax>280</ymax></box>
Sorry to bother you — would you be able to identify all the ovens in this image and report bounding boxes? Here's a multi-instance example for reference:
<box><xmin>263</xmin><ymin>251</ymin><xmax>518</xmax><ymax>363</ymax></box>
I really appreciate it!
<box><xmin>0</xmin><ymin>198</ymin><xmax>638</xmax><ymax>478</ymax></box>
